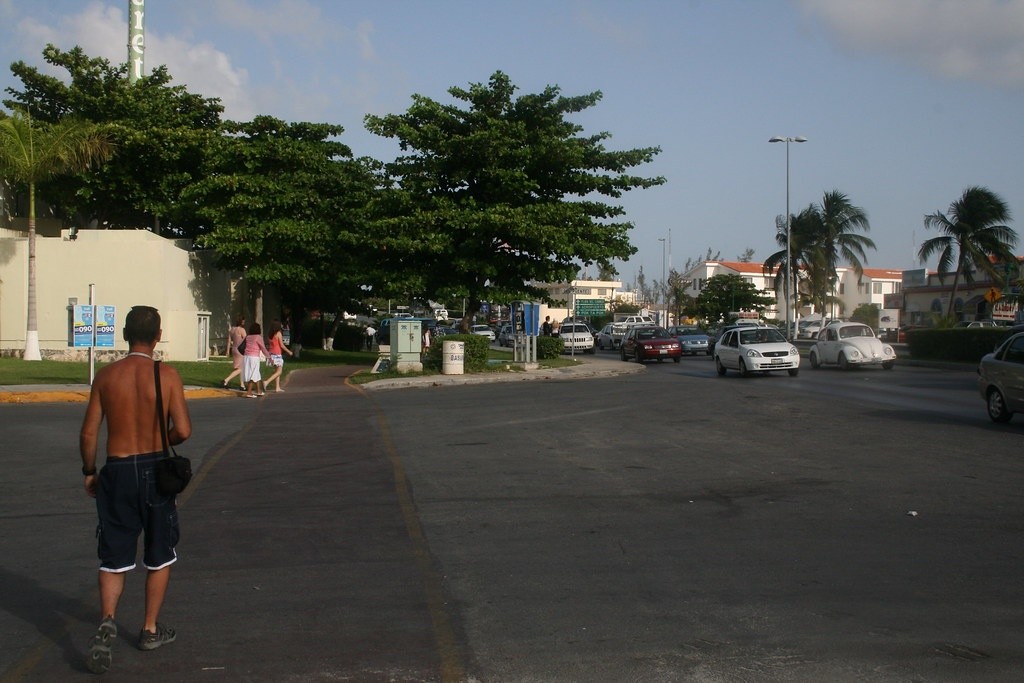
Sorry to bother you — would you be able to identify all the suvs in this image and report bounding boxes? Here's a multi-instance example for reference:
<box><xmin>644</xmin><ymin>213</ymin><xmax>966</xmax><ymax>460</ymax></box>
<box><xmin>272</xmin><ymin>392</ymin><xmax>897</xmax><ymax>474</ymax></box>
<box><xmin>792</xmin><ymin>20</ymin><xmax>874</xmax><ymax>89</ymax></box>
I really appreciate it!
<box><xmin>614</xmin><ymin>316</ymin><xmax>655</xmax><ymax>326</ymax></box>
<box><xmin>498</xmin><ymin>325</ymin><xmax>527</xmax><ymax>347</ymax></box>
<box><xmin>558</xmin><ymin>322</ymin><xmax>594</xmax><ymax>355</ymax></box>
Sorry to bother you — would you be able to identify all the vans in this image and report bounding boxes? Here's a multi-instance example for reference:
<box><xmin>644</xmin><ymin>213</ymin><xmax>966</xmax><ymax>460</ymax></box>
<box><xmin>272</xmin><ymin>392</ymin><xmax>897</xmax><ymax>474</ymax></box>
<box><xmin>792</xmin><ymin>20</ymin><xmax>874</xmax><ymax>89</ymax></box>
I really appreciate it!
<box><xmin>432</xmin><ymin>309</ymin><xmax>448</xmax><ymax>321</ymax></box>
<box><xmin>381</xmin><ymin>317</ymin><xmax>435</xmax><ymax>327</ymax></box>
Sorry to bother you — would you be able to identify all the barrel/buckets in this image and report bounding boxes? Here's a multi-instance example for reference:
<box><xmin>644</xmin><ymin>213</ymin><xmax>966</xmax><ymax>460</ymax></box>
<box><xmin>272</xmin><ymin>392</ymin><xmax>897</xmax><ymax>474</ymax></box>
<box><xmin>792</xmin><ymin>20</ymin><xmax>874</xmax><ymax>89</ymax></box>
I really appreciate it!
<box><xmin>443</xmin><ymin>340</ymin><xmax>465</xmax><ymax>374</ymax></box>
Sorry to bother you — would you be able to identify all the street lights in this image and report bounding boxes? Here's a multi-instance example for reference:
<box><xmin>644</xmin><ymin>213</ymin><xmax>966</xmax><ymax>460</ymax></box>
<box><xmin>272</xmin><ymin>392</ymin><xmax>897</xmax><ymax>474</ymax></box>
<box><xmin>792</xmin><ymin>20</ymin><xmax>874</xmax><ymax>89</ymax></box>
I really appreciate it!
<box><xmin>769</xmin><ymin>135</ymin><xmax>808</xmax><ymax>340</ymax></box>
<box><xmin>658</xmin><ymin>238</ymin><xmax>665</xmax><ymax>328</ymax></box>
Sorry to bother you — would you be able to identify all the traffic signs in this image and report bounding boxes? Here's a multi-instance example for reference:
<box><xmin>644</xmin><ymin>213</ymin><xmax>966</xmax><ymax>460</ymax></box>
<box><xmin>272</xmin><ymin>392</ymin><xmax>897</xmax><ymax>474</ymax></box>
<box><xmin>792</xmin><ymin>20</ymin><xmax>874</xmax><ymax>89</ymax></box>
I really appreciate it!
<box><xmin>575</xmin><ymin>299</ymin><xmax>605</xmax><ymax>317</ymax></box>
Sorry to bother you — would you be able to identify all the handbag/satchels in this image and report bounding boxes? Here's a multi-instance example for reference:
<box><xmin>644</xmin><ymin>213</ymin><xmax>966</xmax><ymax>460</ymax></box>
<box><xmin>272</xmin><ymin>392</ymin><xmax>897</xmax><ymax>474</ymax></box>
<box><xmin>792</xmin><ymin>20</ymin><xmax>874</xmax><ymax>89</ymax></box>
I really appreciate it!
<box><xmin>155</xmin><ymin>456</ymin><xmax>193</xmax><ymax>497</ymax></box>
<box><xmin>237</xmin><ymin>336</ymin><xmax>247</xmax><ymax>355</ymax></box>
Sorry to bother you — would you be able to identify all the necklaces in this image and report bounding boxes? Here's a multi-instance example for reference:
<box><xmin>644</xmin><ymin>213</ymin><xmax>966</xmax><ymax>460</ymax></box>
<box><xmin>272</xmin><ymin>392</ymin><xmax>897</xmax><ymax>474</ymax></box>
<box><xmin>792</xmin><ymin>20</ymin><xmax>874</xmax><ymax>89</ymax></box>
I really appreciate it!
<box><xmin>128</xmin><ymin>351</ymin><xmax>152</xmax><ymax>360</ymax></box>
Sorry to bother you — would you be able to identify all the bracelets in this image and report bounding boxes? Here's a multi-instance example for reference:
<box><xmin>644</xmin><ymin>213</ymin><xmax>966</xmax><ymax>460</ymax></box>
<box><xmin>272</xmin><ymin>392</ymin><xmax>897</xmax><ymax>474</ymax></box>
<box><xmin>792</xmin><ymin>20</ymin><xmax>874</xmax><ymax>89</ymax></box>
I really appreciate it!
<box><xmin>81</xmin><ymin>465</ymin><xmax>97</xmax><ymax>476</ymax></box>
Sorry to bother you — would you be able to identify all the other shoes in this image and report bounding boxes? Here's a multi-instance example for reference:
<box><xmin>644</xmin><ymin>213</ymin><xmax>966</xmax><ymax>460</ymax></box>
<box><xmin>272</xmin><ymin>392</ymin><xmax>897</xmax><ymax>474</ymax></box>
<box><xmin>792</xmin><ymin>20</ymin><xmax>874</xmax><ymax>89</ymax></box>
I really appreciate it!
<box><xmin>247</xmin><ymin>394</ymin><xmax>257</xmax><ymax>398</ymax></box>
<box><xmin>257</xmin><ymin>392</ymin><xmax>264</xmax><ymax>395</ymax></box>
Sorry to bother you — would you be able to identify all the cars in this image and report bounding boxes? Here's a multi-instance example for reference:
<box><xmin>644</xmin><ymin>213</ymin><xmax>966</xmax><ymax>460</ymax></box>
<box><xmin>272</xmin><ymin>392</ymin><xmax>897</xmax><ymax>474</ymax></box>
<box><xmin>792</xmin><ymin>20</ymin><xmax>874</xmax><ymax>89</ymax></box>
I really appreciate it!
<box><xmin>808</xmin><ymin>322</ymin><xmax>896</xmax><ymax>371</ymax></box>
<box><xmin>714</xmin><ymin>327</ymin><xmax>800</xmax><ymax>377</ymax></box>
<box><xmin>668</xmin><ymin>326</ymin><xmax>711</xmax><ymax>356</ymax></box>
<box><xmin>891</xmin><ymin>325</ymin><xmax>926</xmax><ymax>344</ymax></box>
<box><xmin>590</xmin><ymin>322</ymin><xmax>624</xmax><ymax>350</ymax></box>
<box><xmin>620</xmin><ymin>325</ymin><xmax>681</xmax><ymax>363</ymax></box>
<box><xmin>470</xmin><ymin>324</ymin><xmax>496</xmax><ymax>342</ymax></box>
<box><xmin>436</xmin><ymin>317</ymin><xmax>462</xmax><ymax>329</ymax></box>
<box><xmin>282</xmin><ymin>329</ymin><xmax>291</xmax><ymax>346</ymax></box>
<box><xmin>761</xmin><ymin>320</ymin><xmax>843</xmax><ymax>340</ymax></box>
<box><xmin>978</xmin><ymin>330</ymin><xmax>1024</xmax><ymax>422</ymax></box>
<box><xmin>953</xmin><ymin>320</ymin><xmax>997</xmax><ymax>328</ymax></box>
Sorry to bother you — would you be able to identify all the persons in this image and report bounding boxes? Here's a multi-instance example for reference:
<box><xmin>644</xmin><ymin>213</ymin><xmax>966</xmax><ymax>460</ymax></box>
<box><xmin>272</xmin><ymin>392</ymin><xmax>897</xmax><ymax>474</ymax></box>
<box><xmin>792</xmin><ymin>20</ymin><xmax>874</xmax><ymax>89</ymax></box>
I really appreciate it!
<box><xmin>366</xmin><ymin>324</ymin><xmax>375</xmax><ymax>352</ymax></box>
<box><xmin>221</xmin><ymin>315</ymin><xmax>248</xmax><ymax>390</ymax></box>
<box><xmin>422</xmin><ymin>326</ymin><xmax>431</xmax><ymax>358</ymax></box>
<box><xmin>262</xmin><ymin>317</ymin><xmax>293</xmax><ymax>392</ymax></box>
<box><xmin>542</xmin><ymin>316</ymin><xmax>560</xmax><ymax>337</ymax></box>
<box><xmin>242</xmin><ymin>322</ymin><xmax>275</xmax><ymax>398</ymax></box>
<box><xmin>79</xmin><ymin>306</ymin><xmax>192</xmax><ymax>675</ymax></box>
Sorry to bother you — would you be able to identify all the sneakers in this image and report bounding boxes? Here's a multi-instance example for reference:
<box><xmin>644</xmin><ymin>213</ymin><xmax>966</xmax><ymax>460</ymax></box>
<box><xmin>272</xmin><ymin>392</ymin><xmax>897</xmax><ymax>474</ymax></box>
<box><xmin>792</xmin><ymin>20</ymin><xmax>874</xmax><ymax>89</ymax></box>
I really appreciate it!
<box><xmin>137</xmin><ymin>622</ymin><xmax>176</xmax><ymax>650</ymax></box>
<box><xmin>86</xmin><ymin>614</ymin><xmax>118</xmax><ymax>673</ymax></box>
